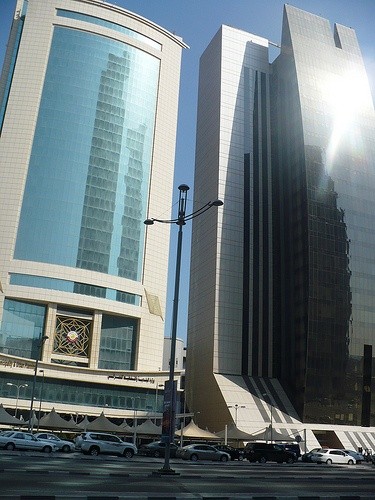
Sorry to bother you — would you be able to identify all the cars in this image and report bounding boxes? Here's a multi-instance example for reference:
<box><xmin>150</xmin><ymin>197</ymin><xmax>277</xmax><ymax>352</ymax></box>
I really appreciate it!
<box><xmin>212</xmin><ymin>445</ymin><xmax>246</xmax><ymax>460</ymax></box>
<box><xmin>311</xmin><ymin>449</ymin><xmax>356</xmax><ymax>465</ymax></box>
<box><xmin>0</xmin><ymin>431</ymin><xmax>59</xmax><ymax>453</ymax></box>
<box><xmin>301</xmin><ymin>448</ymin><xmax>321</xmax><ymax>462</ymax></box>
<box><xmin>342</xmin><ymin>449</ymin><xmax>365</xmax><ymax>462</ymax></box>
<box><xmin>228</xmin><ymin>404</ymin><xmax>246</xmax><ymax>445</ymax></box>
<box><xmin>34</xmin><ymin>433</ymin><xmax>76</xmax><ymax>453</ymax></box>
<box><xmin>175</xmin><ymin>444</ymin><xmax>232</xmax><ymax>463</ymax></box>
<box><xmin>142</xmin><ymin>440</ymin><xmax>182</xmax><ymax>459</ymax></box>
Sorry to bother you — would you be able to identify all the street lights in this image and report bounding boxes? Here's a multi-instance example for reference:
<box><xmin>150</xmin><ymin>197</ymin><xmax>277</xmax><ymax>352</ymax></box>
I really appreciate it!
<box><xmin>6</xmin><ymin>382</ymin><xmax>29</xmax><ymax>417</ymax></box>
<box><xmin>27</xmin><ymin>332</ymin><xmax>49</xmax><ymax>433</ymax></box>
<box><xmin>37</xmin><ymin>370</ymin><xmax>45</xmax><ymax>433</ymax></box>
<box><xmin>142</xmin><ymin>184</ymin><xmax>225</xmax><ymax>474</ymax></box>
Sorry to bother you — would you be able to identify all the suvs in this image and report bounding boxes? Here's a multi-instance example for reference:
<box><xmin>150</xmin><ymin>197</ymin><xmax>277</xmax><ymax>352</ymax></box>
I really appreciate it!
<box><xmin>243</xmin><ymin>443</ymin><xmax>300</xmax><ymax>463</ymax></box>
<box><xmin>76</xmin><ymin>432</ymin><xmax>138</xmax><ymax>460</ymax></box>
